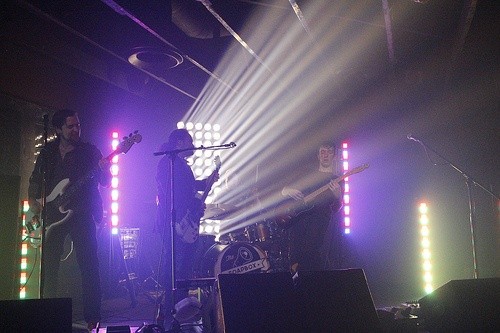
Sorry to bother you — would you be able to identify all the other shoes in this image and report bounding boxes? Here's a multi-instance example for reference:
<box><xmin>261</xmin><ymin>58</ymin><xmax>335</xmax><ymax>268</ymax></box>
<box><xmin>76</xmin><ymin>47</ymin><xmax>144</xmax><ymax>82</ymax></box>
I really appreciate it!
<box><xmin>88</xmin><ymin>320</ymin><xmax>99</xmax><ymax>332</ymax></box>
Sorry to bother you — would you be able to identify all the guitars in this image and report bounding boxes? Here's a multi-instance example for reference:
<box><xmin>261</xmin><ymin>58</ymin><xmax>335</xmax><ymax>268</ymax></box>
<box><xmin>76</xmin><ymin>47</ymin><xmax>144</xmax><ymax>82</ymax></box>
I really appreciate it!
<box><xmin>23</xmin><ymin>129</ymin><xmax>144</xmax><ymax>249</ymax></box>
<box><xmin>274</xmin><ymin>162</ymin><xmax>375</xmax><ymax>229</ymax></box>
<box><xmin>173</xmin><ymin>154</ymin><xmax>223</xmax><ymax>244</ymax></box>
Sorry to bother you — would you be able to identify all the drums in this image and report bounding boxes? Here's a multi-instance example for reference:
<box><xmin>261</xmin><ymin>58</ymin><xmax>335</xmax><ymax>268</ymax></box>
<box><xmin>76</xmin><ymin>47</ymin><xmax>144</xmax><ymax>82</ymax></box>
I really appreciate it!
<box><xmin>202</xmin><ymin>183</ymin><xmax>277</xmax><ymax>244</ymax></box>
<box><xmin>244</xmin><ymin>220</ymin><xmax>278</xmax><ymax>248</ymax></box>
<box><xmin>199</xmin><ymin>241</ymin><xmax>271</xmax><ymax>282</ymax></box>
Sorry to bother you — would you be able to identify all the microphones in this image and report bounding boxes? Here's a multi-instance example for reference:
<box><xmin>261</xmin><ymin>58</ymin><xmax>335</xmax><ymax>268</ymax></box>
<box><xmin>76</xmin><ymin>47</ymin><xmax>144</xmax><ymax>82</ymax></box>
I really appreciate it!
<box><xmin>230</xmin><ymin>142</ymin><xmax>236</xmax><ymax>146</ymax></box>
<box><xmin>42</xmin><ymin>115</ymin><xmax>49</xmax><ymax>137</ymax></box>
<box><xmin>407</xmin><ymin>136</ymin><xmax>422</xmax><ymax>143</ymax></box>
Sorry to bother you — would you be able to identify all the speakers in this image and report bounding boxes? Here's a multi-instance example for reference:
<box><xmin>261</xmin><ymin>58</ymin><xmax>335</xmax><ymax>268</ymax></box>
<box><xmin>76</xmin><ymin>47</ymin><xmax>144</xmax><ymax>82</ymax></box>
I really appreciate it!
<box><xmin>398</xmin><ymin>277</ymin><xmax>500</xmax><ymax>333</ymax></box>
<box><xmin>202</xmin><ymin>268</ymin><xmax>383</xmax><ymax>333</ymax></box>
<box><xmin>0</xmin><ymin>298</ymin><xmax>73</xmax><ymax>333</ymax></box>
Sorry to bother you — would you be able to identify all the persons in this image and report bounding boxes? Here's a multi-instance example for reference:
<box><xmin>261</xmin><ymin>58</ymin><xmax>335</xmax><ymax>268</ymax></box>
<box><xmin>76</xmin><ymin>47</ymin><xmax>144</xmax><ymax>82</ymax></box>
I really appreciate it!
<box><xmin>154</xmin><ymin>128</ymin><xmax>220</xmax><ymax>290</ymax></box>
<box><xmin>281</xmin><ymin>141</ymin><xmax>345</xmax><ymax>275</ymax></box>
<box><xmin>26</xmin><ymin>109</ymin><xmax>113</xmax><ymax>333</ymax></box>
